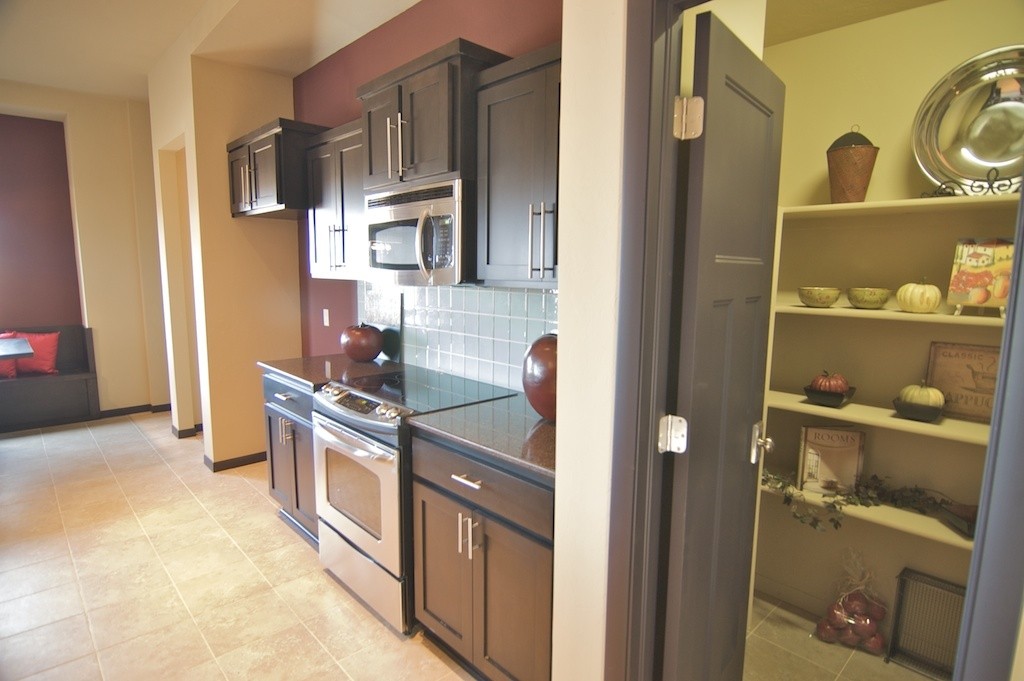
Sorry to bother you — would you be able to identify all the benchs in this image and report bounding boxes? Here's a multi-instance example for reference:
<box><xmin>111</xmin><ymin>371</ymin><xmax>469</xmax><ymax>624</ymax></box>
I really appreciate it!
<box><xmin>0</xmin><ymin>324</ymin><xmax>101</xmax><ymax>434</ymax></box>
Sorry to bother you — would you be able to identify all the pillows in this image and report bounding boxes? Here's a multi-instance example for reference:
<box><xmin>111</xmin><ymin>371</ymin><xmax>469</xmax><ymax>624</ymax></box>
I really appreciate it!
<box><xmin>13</xmin><ymin>332</ymin><xmax>60</xmax><ymax>375</ymax></box>
<box><xmin>0</xmin><ymin>330</ymin><xmax>19</xmax><ymax>379</ymax></box>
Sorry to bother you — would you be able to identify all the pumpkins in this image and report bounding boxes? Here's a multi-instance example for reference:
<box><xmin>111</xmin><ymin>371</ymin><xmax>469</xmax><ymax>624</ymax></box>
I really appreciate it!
<box><xmin>967</xmin><ymin>276</ymin><xmax>1010</xmax><ymax>304</ymax></box>
<box><xmin>899</xmin><ymin>378</ymin><xmax>945</xmax><ymax>409</ymax></box>
<box><xmin>810</xmin><ymin>369</ymin><xmax>850</xmax><ymax>393</ymax></box>
<box><xmin>896</xmin><ymin>276</ymin><xmax>942</xmax><ymax>313</ymax></box>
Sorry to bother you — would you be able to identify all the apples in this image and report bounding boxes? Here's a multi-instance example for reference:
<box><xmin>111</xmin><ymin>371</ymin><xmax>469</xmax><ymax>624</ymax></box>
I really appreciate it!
<box><xmin>817</xmin><ymin>591</ymin><xmax>886</xmax><ymax>656</ymax></box>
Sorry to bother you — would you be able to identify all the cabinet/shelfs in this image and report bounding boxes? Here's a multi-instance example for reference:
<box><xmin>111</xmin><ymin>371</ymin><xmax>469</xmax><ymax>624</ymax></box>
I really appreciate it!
<box><xmin>407</xmin><ymin>427</ymin><xmax>555</xmax><ymax>681</ymax></box>
<box><xmin>262</xmin><ymin>373</ymin><xmax>320</xmax><ymax>553</ymax></box>
<box><xmin>473</xmin><ymin>37</ymin><xmax>562</xmax><ymax>289</ymax></box>
<box><xmin>361</xmin><ymin>36</ymin><xmax>513</xmax><ymax>191</ymax></box>
<box><xmin>227</xmin><ymin>116</ymin><xmax>332</xmax><ymax>219</ymax></box>
<box><xmin>303</xmin><ymin>117</ymin><xmax>370</xmax><ymax>281</ymax></box>
<box><xmin>739</xmin><ymin>192</ymin><xmax>1023</xmax><ymax>635</ymax></box>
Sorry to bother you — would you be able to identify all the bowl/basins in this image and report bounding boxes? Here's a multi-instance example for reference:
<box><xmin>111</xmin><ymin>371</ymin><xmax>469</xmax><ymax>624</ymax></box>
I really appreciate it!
<box><xmin>797</xmin><ymin>286</ymin><xmax>844</xmax><ymax>308</ymax></box>
<box><xmin>892</xmin><ymin>397</ymin><xmax>949</xmax><ymax>422</ymax></box>
<box><xmin>802</xmin><ymin>384</ymin><xmax>856</xmax><ymax>409</ymax></box>
<box><xmin>847</xmin><ymin>287</ymin><xmax>894</xmax><ymax>309</ymax></box>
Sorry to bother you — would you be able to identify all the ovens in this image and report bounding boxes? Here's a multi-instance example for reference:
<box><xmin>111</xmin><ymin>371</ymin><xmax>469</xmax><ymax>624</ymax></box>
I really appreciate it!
<box><xmin>312</xmin><ymin>411</ymin><xmax>415</xmax><ymax>636</ymax></box>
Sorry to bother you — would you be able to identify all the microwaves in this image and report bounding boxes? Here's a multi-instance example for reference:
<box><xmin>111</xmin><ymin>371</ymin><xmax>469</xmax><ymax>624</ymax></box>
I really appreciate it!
<box><xmin>364</xmin><ymin>178</ymin><xmax>478</xmax><ymax>289</ymax></box>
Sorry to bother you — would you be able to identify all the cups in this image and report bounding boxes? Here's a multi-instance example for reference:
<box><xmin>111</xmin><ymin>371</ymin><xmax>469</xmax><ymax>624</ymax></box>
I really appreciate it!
<box><xmin>826</xmin><ymin>124</ymin><xmax>880</xmax><ymax>203</ymax></box>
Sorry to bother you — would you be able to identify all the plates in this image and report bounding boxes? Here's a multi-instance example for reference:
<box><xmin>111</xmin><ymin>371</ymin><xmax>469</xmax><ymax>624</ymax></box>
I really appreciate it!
<box><xmin>909</xmin><ymin>44</ymin><xmax>1024</xmax><ymax>194</ymax></box>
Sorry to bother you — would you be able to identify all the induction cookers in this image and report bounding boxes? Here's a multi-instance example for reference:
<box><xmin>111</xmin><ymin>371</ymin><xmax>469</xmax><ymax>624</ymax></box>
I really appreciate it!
<box><xmin>312</xmin><ymin>367</ymin><xmax>519</xmax><ymax>449</ymax></box>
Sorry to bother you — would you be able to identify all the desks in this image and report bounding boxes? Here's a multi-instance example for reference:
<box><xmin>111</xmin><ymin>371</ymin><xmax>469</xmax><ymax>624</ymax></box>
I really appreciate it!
<box><xmin>0</xmin><ymin>337</ymin><xmax>34</xmax><ymax>359</ymax></box>
